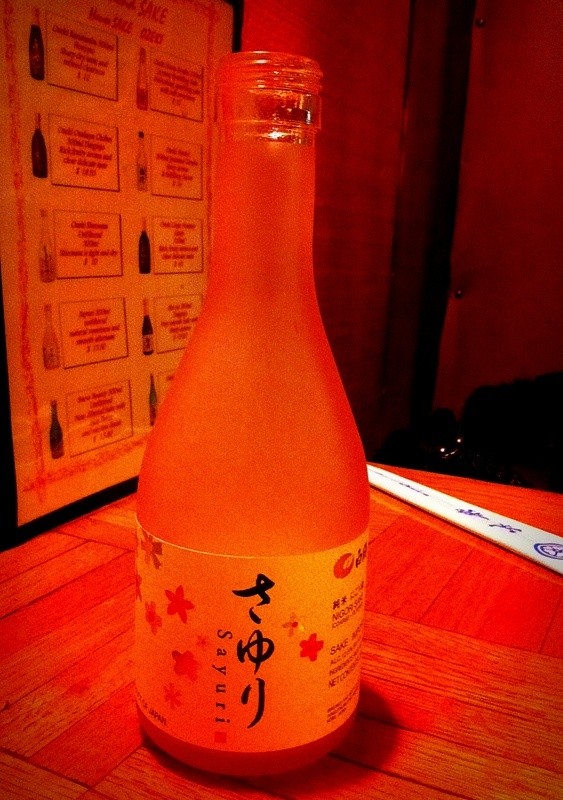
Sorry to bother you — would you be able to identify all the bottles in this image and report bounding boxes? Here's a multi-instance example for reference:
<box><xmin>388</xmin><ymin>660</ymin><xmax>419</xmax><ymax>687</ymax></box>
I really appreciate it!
<box><xmin>141</xmin><ymin>298</ymin><xmax>155</xmax><ymax>355</ymax></box>
<box><xmin>138</xmin><ymin>216</ymin><xmax>150</xmax><ymax>274</ymax></box>
<box><xmin>42</xmin><ymin>304</ymin><xmax>61</xmax><ymax>370</ymax></box>
<box><xmin>28</xmin><ymin>6</ymin><xmax>45</xmax><ymax>81</ymax></box>
<box><xmin>135</xmin><ymin>131</ymin><xmax>149</xmax><ymax>192</ymax></box>
<box><xmin>37</xmin><ymin>208</ymin><xmax>58</xmax><ymax>283</ymax></box>
<box><xmin>31</xmin><ymin>112</ymin><xmax>48</xmax><ymax>178</ymax></box>
<box><xmin>50</xmin><ymin>400</ymin><xmax>65</xmax><ymax>459</ymax></box>
<box><xmin>131</xmin><ymin>51</ymin><xmax>379</xmax><ymax>774</ymax></box>
<box><xmin>136</xmin><ymin>49</ymin><xmax>149</xmax><ymax>111</ymax></box>
<box><xmin>149</xmin><ymin>372</ymin><xmax>159</xmax><ymax>426</ymax></box>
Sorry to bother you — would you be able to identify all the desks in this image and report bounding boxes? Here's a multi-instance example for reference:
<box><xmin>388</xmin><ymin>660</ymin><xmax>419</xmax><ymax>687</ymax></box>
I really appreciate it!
<box><xmin>0</xmin><ymin>460</ymin><xmax>563</xmax><ymax>800</ymax></box>
